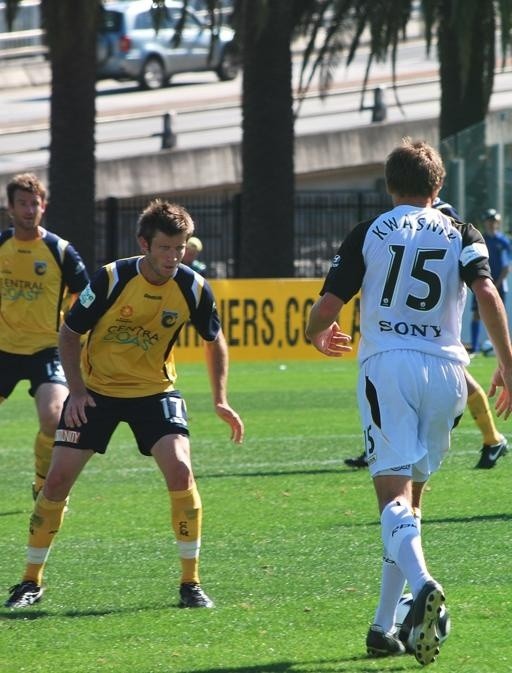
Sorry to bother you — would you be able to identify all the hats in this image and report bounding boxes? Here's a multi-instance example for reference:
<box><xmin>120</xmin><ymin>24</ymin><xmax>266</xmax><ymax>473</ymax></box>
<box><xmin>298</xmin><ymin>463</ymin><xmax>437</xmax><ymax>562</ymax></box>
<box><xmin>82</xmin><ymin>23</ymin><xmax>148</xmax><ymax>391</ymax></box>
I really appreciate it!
<box><xmin>481</xmin><ymin>208</ymin><xmax>500</xmax><ymax>220</ymax></box>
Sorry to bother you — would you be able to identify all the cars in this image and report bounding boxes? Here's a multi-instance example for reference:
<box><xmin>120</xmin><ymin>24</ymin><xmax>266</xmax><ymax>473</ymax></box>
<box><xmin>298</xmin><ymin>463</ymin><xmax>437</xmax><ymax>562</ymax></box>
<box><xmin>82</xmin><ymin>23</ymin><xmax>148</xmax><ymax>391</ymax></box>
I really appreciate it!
<box><xmin>93</xmin><ymin>0</ymin><xmax>244</xmax><ymax>89</ymax></box>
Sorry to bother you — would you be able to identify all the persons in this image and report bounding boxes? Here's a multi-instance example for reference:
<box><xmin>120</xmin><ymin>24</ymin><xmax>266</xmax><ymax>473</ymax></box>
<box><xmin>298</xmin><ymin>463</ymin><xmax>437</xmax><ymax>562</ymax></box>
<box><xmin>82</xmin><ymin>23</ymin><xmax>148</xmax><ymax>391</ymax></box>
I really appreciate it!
<box><xmin>466</xmin><ymin>205</ymin><xmax>510</xmax><ymax>356</ymax></box>
<box><xmin>0</xmin><ymin>171</ymin><xmax>96</xmax><ymax>516</ymax></box>
<box><xmin>177</xmin><ymin>236</ymin><xmax>208</xmax><ymax>279</ymax></box>
<box><xmin>301</xmin><ymin>135</ymin><xmax>511</xmax><ymax>665</ymax></box>
<box><xmin>337</xmin><ymin>195</ymin><xmax>508</xmax><ymax>471</ymax></box>
<box><xmin>1</xmin><ymin>197</ymin><xmax>245</xmax><ymax>612</ymax></box>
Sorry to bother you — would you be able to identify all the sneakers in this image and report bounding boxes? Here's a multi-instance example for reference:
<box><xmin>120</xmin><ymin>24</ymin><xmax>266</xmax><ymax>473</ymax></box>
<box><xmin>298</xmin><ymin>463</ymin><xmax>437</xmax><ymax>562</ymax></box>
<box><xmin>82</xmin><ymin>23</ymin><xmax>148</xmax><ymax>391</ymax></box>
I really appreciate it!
<box><xmin>366</xmin><ymin>624</ymin><xmax>406</xmax><ymax>655</ymax></box>
<box><xmin>344</xmin><ymin>451</ymin><xmax>368</xmax><ymax>467</ymax></box>
<box><xmin>412</xmin><ymin>580</ymin><xmax>445</xmax><ymax>665</ymax></box>
<box><xmin>474</xmin><ymin>438</ymin><xmax>510</xmax><ymax>468</ymax></box>
<box><xmin>5</xmin><ymin>580</ymin><xmax>42</xmax><ymax>608</ymax></box>
<box><xmin>179</xmin><ymin>583</ymin><xmax>213</xmax><ymax>608</ymax></box>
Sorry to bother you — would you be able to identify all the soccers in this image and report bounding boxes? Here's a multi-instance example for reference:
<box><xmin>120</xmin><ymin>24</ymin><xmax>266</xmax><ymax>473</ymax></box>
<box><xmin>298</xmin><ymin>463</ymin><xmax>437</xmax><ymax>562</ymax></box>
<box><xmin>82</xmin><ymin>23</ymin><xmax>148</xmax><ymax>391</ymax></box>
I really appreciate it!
<box><xmin>395</xmin><ymin>592</ymin><xmax>451</xmax><ymax>649</ymax></box>
